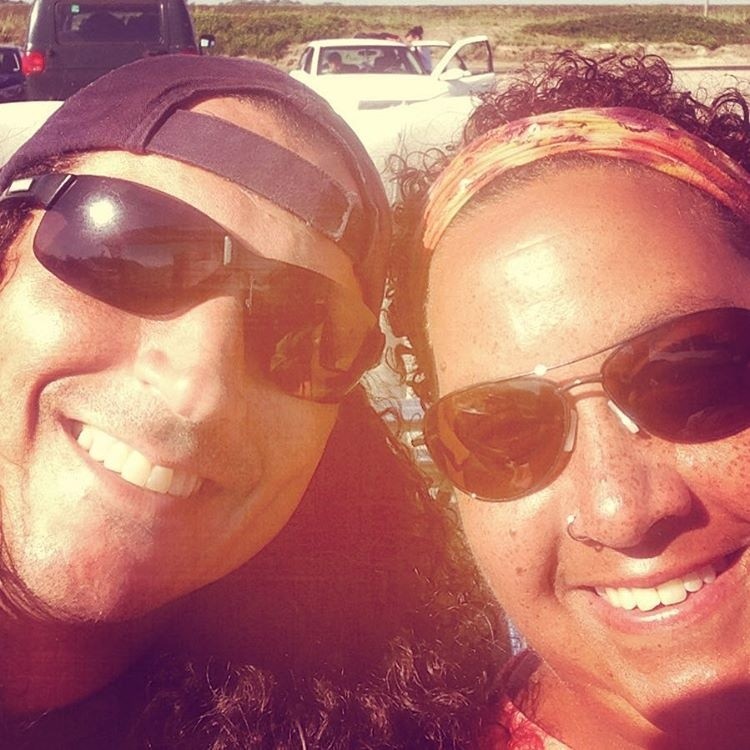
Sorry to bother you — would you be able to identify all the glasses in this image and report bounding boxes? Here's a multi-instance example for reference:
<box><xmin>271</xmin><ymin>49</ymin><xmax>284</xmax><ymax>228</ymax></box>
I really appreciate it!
<box><xmin>412</xmin><ymin>306</ymin><xmax>750</xmax><ymax>503</ymax></box>
<box><xmin>2</xmin><ymin>173</ymin><xmax>385</xmax><ymax>404</ymax></box>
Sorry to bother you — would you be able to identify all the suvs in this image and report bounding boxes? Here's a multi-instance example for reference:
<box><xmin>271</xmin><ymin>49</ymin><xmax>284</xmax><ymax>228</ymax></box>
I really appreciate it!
<box><xmin>22</xmin><ymin>0</ymin><xmax>216</xmax><ymax>104</ymax></box>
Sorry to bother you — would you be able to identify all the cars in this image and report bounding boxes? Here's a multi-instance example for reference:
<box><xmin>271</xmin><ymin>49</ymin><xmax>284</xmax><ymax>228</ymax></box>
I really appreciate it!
<box><xmin>288</xmin><ymin>36</ymin><xmax>497</xmax><ymax>116</ymax></box>
<box><xmin>0</xmin><ymin>44</ymin><xmax>25</xmax><ymax>105</ymax></box>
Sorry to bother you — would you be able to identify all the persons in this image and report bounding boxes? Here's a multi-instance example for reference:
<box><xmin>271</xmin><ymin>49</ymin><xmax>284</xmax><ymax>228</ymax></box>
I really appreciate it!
<box><xmin>404</xmin><ymin>26</ymin><xmax>432</xmax><ymax>75</ymax></box>
<box><xmin>384</xmin><ymin>50</ymin><xmax>749</xmax><ymax>750</ymax></box>
<box><xmin>0</xmin><ymin>51</ymin><xmax>511</xmax><ymax>750</ymax></box>
<box><xmin>321</xmin><ymin>52</ymin><xmax>350</xmax><ymax>74</ymax></box>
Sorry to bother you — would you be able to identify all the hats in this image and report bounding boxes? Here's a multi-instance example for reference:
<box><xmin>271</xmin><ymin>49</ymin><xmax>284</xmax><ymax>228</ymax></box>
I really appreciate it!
<box><xmin>0</xmin><ymin>53</ymin><xmax>391</xmax><ymax>323</ymax></box>
